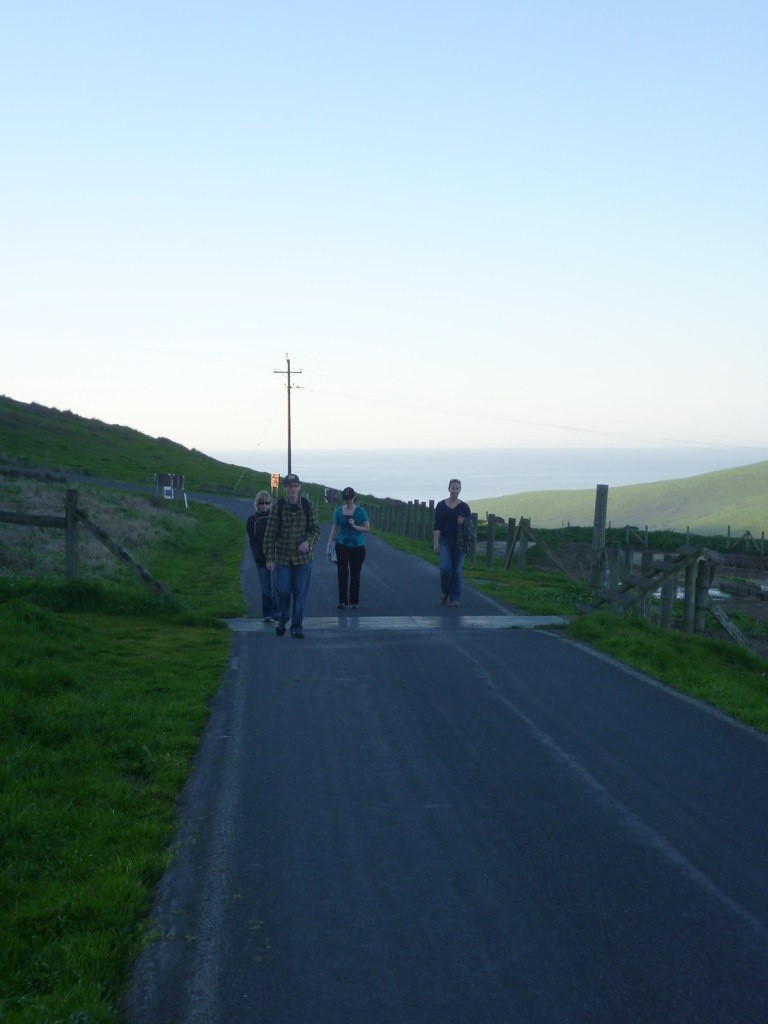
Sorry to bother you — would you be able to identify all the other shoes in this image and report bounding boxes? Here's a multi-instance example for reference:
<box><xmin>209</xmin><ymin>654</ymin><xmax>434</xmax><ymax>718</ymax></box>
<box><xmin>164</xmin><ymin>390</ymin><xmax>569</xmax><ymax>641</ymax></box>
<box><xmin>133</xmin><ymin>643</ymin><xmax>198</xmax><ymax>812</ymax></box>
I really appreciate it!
<box><xmin>351</xmin><ymin>604</ymin><xmax>359</xmax><ymax>609</ymax></box>
<box><xmin>337</xmin><ymin>603</ymin><xmax>348</xmax><ymax>609</ymax></box>
<box><xmin>449</xmin><ymin>601</ymin><xmax>460</xmax><ymax>607</ymax></box>
<box><xmin>276</xmin><ymin>620</ymin><xmax>286</xmax><ymax>636</ymax></box>
<box><xmin>264</xmin><ymin>616</ymin><xmax>275</xmax><ymax>622</ymax></box>
<box><xmin>290</xmin><ymin>627</ymin><xmax>304</xmax><ymax>639</ymax></box>
<box><xmin>441</xmin><ymin>593</ymin><xmax>447</xmax><ymax>605</ymax></box>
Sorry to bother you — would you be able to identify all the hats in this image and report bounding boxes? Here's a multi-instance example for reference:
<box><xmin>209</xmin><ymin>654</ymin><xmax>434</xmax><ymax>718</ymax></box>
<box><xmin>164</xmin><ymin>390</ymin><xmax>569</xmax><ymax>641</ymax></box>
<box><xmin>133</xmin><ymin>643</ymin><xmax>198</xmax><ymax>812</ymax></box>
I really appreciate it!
<box><xmin>284</xmin><ymin>474</ymin><xmax>300</xmax><ymax>486</ymax></box>
<box><xmin>342</xmin><ymin>487</ymin><xmax>356</xmax><ymax>500</ymax></box>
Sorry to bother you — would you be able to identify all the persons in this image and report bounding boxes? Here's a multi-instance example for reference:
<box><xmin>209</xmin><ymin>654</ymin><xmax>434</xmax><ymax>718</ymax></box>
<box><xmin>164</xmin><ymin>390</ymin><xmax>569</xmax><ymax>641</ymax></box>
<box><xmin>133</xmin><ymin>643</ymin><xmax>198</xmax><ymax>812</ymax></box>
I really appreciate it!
<box><xmin>247</xmin><ymin>490</ymin><xmax>274</xmax><ymax>623</ymax></box>
<box><xmin>327</xmin><ymin>487</ymin><xmax>370</xmax><ymax>609</ymax></box>
<box><xmin>262</xmin><ymin>474</ymin><xmax>321</xmax><ymax>638</ymax></box>
<box><xmin>433</xmin><ymin>479</ymin><xmax>472</xmax><ymax>606</ymax></box>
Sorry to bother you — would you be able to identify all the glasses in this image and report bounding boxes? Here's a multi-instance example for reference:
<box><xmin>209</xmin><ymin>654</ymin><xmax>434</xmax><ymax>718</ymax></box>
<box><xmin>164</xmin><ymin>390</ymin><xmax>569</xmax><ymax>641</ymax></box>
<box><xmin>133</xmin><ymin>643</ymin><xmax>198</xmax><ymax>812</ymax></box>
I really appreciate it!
<box><xmin>259</xmin><ymin>501</ymin><xmax>271</xmax><ymax>505</ymax></box>
